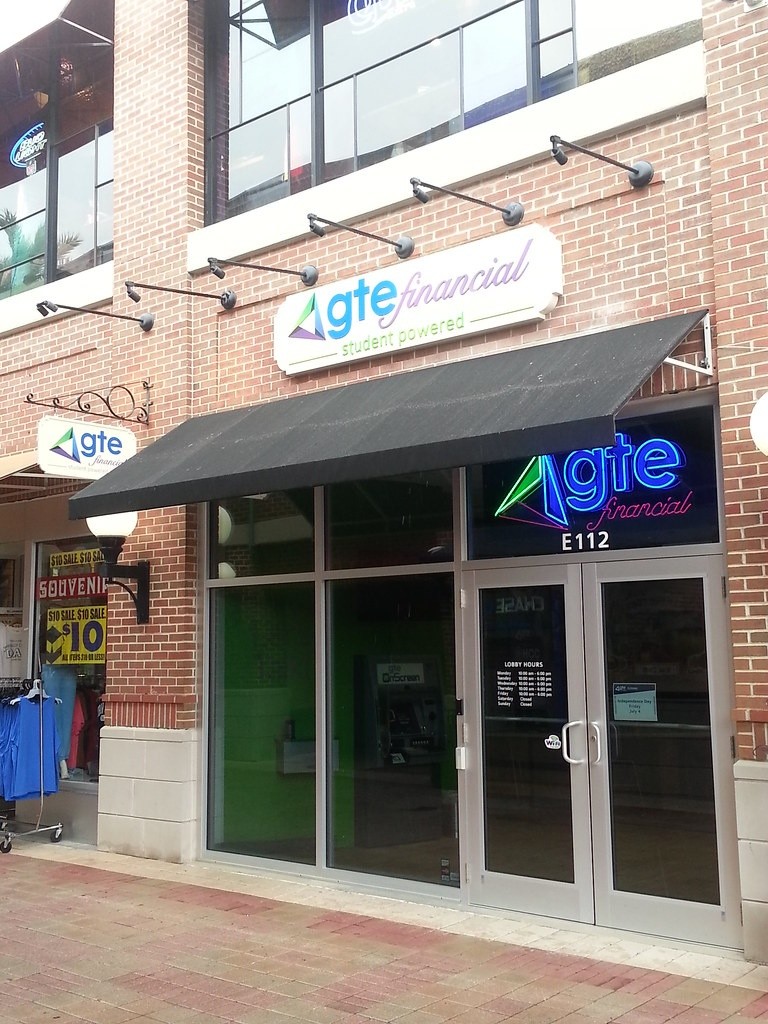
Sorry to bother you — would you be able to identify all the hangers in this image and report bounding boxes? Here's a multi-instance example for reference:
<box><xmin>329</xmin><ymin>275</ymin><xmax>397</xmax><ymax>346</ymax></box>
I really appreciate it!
<box><xmin>0</xmin><ymin>677</ymin><xmax>63</xmax><ymax>706</ymax></box>
<box><xmin>0</xmin><ymin>614</ymin><xmax>22</xmax><ymax>626</ymax></box>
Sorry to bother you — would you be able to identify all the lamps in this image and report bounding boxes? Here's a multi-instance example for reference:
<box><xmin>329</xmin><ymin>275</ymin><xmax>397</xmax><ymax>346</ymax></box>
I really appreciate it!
<box><xmin>85</xmin><ymin>511</ymin><xmax>150</xmax><ymax>626</ymax></box>
